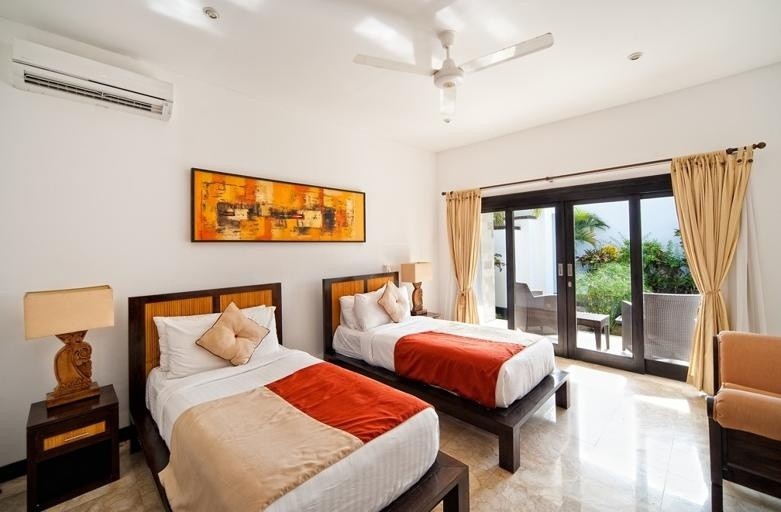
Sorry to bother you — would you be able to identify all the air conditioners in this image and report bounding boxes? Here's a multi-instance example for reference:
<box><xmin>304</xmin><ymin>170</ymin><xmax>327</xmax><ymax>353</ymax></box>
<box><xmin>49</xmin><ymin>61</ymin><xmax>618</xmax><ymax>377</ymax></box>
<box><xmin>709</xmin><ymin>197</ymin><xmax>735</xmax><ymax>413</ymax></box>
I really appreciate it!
<box><xmin>9</xmin><ymin>36</ymin><xmax>176</xmax><ymax>124</ymax></box>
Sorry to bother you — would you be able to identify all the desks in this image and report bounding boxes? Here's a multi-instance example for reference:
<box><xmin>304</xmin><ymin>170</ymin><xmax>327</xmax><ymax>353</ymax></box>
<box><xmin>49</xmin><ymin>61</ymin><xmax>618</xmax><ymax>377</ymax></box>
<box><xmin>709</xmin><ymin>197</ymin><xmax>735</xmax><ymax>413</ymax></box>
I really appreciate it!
<box><xmin>575</xmin><ymin>311</ymin><xmax>611</xmax><ymax>352</ymax></box>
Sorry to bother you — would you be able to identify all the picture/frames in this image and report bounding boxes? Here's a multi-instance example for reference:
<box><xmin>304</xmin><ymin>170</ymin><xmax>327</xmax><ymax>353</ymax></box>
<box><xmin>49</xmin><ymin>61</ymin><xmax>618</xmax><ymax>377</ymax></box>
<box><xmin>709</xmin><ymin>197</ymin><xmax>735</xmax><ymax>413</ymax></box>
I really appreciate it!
<box><xmin>190</xmin><ymin>166</ymin><xmax>368</xmax><ymax>244</ymax></box>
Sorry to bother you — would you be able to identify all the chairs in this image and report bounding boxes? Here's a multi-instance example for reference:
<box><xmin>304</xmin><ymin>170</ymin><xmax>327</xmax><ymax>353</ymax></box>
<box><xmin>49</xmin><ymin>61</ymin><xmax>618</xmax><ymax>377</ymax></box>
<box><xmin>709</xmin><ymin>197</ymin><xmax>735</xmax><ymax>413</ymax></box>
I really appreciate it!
<box><xmin>706</xmin><ymin>329</ymin><xmax>781</xmax><ymax>511</ymax></box>
<box><xmin>515</xmin><ymin>278</ymin><xmax>558</xmax><ymax>335</ymax></box>
<box><xmin>621</xmin><ymin>292</ymin><xmax>702</xmax><ymax>362</ymax></box>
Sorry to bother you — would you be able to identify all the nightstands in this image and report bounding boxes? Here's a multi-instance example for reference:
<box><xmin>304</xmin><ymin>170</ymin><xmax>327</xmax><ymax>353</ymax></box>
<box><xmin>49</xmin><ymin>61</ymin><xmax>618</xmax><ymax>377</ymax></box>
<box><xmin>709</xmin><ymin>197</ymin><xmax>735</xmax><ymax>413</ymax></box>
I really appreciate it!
<box><xmin>26</xmin><ymin>382</ymin><xmax>121</xmax><ymax>512</ymax></box>
<box><xmin>411</xmin><ymin>309</ymin><xmax>440</xmax><ymax>319</ymax></box>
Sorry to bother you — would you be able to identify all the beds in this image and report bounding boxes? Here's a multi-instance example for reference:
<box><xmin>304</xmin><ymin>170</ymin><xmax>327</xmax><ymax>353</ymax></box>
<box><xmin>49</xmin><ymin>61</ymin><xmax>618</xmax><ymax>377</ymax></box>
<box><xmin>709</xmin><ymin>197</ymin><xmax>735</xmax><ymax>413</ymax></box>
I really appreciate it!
<box><xmin>128</xmin><ymin>281</ymin><xmax>473</xmax><ymax>512</ymax></box>
<box><xmin>322</xmin><ymin>271</ymin><xmax>571</xmax><ymax>474</ymax></box>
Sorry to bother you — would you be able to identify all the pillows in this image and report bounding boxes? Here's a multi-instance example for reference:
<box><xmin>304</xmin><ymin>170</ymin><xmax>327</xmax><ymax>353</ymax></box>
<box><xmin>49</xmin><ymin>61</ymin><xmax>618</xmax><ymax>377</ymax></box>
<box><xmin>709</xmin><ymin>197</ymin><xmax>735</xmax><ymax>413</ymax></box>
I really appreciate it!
<box><xmin>152</xmin><ymin>303</ymin><xmax>267</xmax><ymax>373</ymax></box>
<box><xmin>353</xmin><ymin>283</ymin><xmax>393</xmax><ymax>331</ymax></box>
<box><xmin>337</xmin><ymin>294</ymin><xmax>359</xmax><ymax>331</ymax></box>
<box><xmin>377</xmin><ymin>278</ymin><xmax>410</xmax><ymax>324</ymax></box>
<box><xmin>162</xmin><ymin>305</ymin><xmax>280</xmax><ymax>381</ymax></box>
<box><xmin>196</xmin><ymin>299</ymin><xmax>271</xmax><ymax>369</ymax></box>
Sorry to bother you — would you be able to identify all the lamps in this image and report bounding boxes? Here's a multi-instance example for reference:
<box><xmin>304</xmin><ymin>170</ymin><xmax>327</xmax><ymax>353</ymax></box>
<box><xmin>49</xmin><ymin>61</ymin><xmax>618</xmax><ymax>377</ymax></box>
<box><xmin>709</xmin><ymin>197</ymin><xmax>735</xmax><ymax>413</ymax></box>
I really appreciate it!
<box><xmin>20</xmin><ymin>283</ymin><xmax>117</xmax><ymax>408</ymax></box>
<box><xmin>401</xmin><ymin>261</ymin><xmax>434</xmax><ymax>316</ymax></box>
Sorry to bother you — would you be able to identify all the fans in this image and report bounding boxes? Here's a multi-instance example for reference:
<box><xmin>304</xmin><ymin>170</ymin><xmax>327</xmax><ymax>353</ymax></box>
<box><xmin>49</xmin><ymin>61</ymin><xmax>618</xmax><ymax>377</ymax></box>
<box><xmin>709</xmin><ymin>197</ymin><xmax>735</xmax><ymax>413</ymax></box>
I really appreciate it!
<box><xmin>353</xmin><ymin>28</ymin><xmax>555</xmax><ymax>90</ymax></box>
